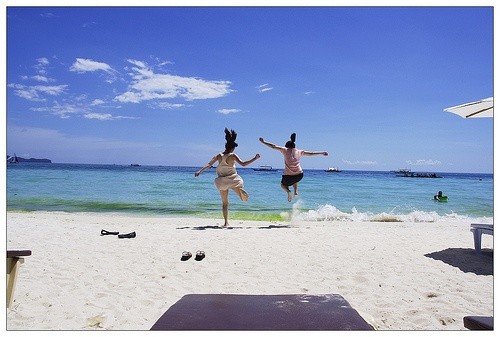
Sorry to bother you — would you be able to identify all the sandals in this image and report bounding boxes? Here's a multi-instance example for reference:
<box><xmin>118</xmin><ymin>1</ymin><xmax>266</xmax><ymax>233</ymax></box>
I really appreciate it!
<box><xmin>196</xmin><ymin>250</ymin><xmax>205</xmax><ymax>260</ymax></box>
<box><xmin>182</xmin><ymin>251</ymin><xmax>192</xmax><ymax>260</ymax></box>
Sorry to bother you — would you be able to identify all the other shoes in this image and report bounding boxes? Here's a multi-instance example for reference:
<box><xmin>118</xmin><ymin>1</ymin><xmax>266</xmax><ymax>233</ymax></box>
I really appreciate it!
<box><xmin>118</xmin><ymin>232</ymin><xmax>136</xmax><ymax>239</ymax></box>
<box><xmin>101</xmin><ymin>229</ymin><xmax>119</xmax><ymax>235</ymax></box>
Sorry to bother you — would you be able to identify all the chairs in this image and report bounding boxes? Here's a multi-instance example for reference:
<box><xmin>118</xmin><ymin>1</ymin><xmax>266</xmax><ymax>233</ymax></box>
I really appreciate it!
<box><xmin>150</xmin><ymin>294</ymin><xmax>377</xmax><ymax>330</ymax></box>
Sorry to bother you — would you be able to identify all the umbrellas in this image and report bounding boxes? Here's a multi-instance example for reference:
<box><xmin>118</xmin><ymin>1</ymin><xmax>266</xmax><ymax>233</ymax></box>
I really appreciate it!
<box><xmin>443</xmin><ymin>97</ymin><xmax>494</xmax><ymax>119</ymax></box>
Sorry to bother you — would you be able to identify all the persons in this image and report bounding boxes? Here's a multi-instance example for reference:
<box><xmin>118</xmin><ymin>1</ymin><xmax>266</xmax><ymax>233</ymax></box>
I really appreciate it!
<box><xmin>195</xmin><ymin>128</ymin><xmax>261</xmax><ymax>226</ymax></box>
<box><xmin>258</xmin><ymin>133</ymin><xmax>329</xmax><ymax>201</ymax></box>
<box><xmin>432</xmin><ymin>191</ymin><xmax>443</xmax><ymax>200</ymax></box>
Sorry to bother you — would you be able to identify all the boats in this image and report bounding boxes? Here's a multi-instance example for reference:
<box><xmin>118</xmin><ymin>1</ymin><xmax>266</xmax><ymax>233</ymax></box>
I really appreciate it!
<box><xmin>323</xmin><ymin>167</ymin><xmax>344</xmax><ymax>173</ymax></box>
<box><xmin>434</xmin><ymin>194</ymin><xmax>449</xmax><ymax>203</ymax></box>
<box><xmin>389</xmin><ymin>168</ymin><xmax>443</xmax><ymax>178</ymax></box>
<box><xmin>251</xmin><ymin>166</ymin><xmax>280</xmax><ymax>171</ymax></box>
<box><xmin>128</xmin><ymin>163</ymin><xmax>142</xmax><ymax>167</ymax></box>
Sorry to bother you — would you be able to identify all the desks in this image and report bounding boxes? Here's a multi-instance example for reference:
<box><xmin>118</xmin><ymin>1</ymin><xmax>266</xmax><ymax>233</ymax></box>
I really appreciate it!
<box><xmin>470</xmin><ymin>224</ymin><xmax>494</xmax><ymax>257</ymax></box>
<box><xmin>7</xmin><ymin>250</ymin><xmax>31</xmax><ymax>307</ymax></box>
